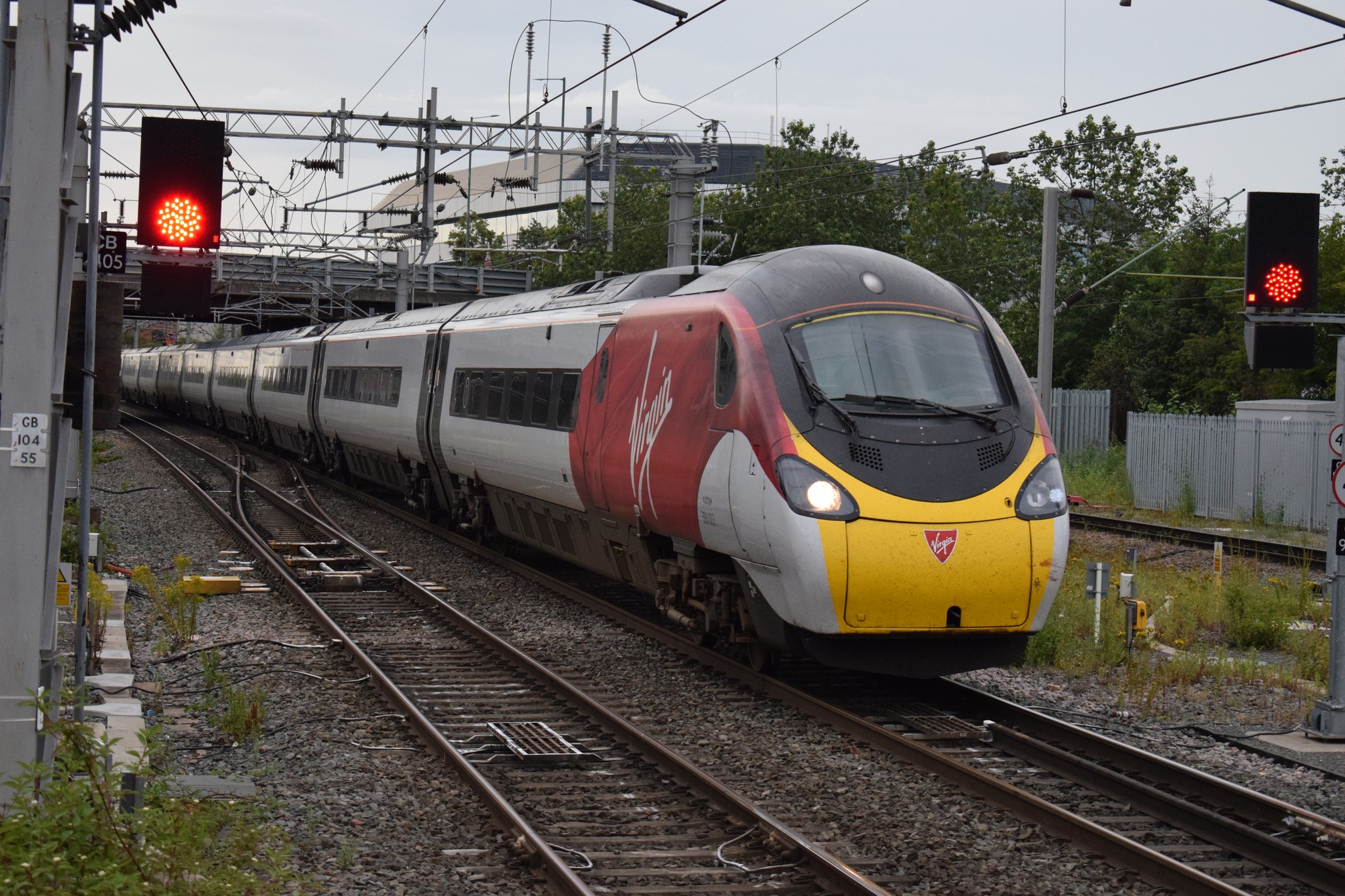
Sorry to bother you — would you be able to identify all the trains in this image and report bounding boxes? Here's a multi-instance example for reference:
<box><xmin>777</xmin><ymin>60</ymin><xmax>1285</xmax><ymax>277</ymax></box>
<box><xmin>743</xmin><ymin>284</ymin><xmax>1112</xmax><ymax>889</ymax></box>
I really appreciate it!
<box><xmin>116</xmin><ymin>244</ymin><xmax>1075</xmax><ymax>678</ymax></box>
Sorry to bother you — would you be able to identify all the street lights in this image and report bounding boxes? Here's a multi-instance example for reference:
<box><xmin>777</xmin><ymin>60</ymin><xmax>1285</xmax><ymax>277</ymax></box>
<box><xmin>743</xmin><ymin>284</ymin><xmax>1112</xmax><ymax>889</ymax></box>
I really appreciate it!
<box><xmin>1040</xmin><ymin>186</ymin><xmax>1099</xmax><ymax>432</ymax></box>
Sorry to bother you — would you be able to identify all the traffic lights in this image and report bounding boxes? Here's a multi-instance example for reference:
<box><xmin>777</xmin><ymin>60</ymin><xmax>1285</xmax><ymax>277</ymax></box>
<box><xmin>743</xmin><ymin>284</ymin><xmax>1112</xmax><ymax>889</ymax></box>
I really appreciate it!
<box><xmin>137</xmin><ymin>115</ymin><xmax>225</xmax><ymax>249</ymax></box>
<box><xmin>1242</xmin><ymin>321</ymin><xmax>1318</xmax><ymax>370</ymax></box>
<box><xmin>139</xmin><ymin>263</ymin><xmax>212</xmax><ymax>316</ymax></box>
<box><xmin>1243</xmin><ymin>192</ymin><xmax>1319</xmax><ymax>309</ymax></box>
